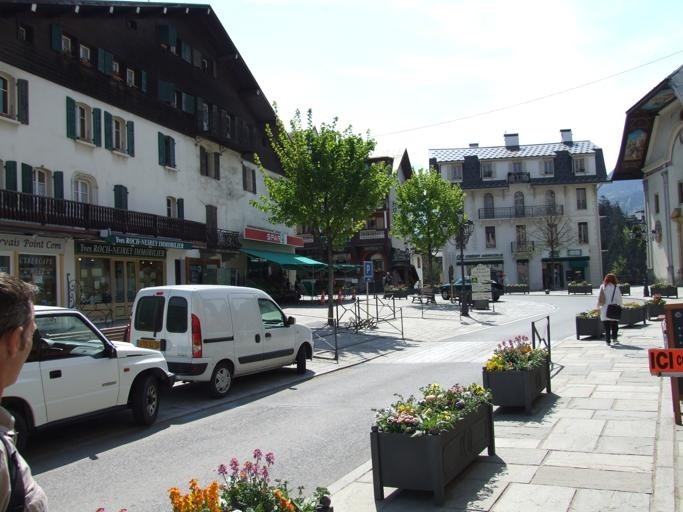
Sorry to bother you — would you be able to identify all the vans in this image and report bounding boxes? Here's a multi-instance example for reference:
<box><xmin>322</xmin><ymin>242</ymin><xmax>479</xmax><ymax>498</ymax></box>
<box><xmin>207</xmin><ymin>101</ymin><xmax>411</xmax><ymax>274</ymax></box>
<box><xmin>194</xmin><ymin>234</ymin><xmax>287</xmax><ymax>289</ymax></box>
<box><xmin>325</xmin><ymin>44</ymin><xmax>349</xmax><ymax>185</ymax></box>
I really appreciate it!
<box><xmin>129</xmin><ymin>285</ymin><xmax>314</xmax><ymax>399</ymax></box>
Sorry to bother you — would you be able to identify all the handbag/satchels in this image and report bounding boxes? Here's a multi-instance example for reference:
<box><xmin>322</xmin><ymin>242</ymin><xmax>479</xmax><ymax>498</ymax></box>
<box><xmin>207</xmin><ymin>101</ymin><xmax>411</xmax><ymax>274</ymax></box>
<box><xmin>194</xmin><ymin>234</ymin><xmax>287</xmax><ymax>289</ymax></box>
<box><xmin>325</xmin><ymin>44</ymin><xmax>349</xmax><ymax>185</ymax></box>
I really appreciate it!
<box><xmin>607</xmin><ymin>304</ymin><xmax>622</xmax><ymax>320</ymax></box>
<box><xmin>598</xmin><ymin>286</ymin><xmax>605</xmax><ymax>306</ymax></box>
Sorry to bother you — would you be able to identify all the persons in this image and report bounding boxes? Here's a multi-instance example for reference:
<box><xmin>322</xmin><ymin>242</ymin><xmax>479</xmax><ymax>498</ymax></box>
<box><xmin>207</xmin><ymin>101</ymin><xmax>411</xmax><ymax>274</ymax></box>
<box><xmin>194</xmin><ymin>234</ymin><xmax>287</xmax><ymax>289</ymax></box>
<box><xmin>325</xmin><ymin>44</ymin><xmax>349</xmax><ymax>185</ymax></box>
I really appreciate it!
<box><xmin>596</xmin><ymin>273</ymin><xmax>624</xmax><ymax>347</ymax></box>
<box><xmin>381</xmin><ymin>271</ymin><xmax>393</xmax><ymax>300</ymax></box>
<box><xmin>1</xmin><ymin>271</ymin><xmax>51</xmax><ymax>512</ymax></box>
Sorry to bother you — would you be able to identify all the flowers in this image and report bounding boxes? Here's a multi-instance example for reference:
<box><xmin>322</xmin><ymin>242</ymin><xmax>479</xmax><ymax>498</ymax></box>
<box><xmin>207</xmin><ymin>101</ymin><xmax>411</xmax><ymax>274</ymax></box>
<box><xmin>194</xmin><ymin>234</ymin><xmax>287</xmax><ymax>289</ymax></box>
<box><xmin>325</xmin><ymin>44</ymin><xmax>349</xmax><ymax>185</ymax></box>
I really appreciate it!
<box><xmin>94</xmin><ymin>447</ymin><xmax>333</xmax><ymax>512</ymax></box>
<box><xmin>370</xmin><ymin>332</ymin><xmax>552</xmax><ymax>436</ymax></box>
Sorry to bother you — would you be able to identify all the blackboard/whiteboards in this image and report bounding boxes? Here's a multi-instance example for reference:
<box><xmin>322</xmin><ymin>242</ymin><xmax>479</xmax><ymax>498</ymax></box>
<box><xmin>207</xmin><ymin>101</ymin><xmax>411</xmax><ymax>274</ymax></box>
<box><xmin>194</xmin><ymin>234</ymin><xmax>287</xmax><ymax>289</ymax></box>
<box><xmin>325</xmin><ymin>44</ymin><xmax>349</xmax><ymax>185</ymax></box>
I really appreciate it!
<box><xmin>664</xmin><ymin>303</ymin><xmax>683</xmax><ymax>399</ymax></box>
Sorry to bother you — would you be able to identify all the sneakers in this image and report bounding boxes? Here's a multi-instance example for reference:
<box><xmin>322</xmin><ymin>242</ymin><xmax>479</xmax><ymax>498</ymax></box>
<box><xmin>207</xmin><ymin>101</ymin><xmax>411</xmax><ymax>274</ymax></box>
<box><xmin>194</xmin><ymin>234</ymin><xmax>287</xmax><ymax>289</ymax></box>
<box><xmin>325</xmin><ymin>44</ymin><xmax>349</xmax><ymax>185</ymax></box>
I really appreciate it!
<box><xmin>606</xmin><ymin>340</ymin><xmax>619</xmax><ymax>345</ymax></box>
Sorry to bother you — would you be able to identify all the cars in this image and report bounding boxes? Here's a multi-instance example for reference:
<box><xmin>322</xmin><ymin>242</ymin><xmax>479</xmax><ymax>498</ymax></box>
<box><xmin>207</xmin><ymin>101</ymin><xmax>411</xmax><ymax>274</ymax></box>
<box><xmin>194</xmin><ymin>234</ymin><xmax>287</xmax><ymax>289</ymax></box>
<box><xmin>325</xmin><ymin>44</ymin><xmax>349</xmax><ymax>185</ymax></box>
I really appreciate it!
<box><xmin>1</xmin><ymin>302</ymin><xmax>171</xmax><ymax>434</ymax></box>
<box><xmin>438</xmin><ymin>275</ymin><xmax>505</xmax><ymax>302</ymax></box>
